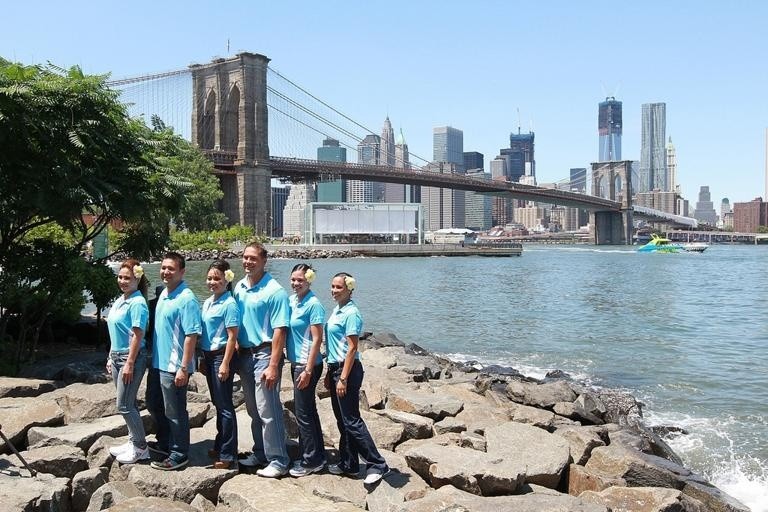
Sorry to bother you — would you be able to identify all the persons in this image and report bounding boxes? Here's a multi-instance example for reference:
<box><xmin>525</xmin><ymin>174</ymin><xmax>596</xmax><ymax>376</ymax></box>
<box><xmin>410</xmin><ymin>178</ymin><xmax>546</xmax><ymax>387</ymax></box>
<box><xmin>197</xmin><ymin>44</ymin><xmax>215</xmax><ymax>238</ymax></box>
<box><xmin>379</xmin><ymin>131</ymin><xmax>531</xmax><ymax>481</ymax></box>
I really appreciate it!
<box><xmin>286</xmin><ymin>264</ymin><xmax>328</xmax><ymax>477</ymax></box>
<box><xmin>233</xmin><ymin>242</ymin><xmax>290</xmax><ymax>478</ymax></box>
<box><xmin>148</xmin><ymin>285</ymin><xmax>162</xmax><ymax>326</ymax></box>
<box><xmin>106</xmin><ymin>260</ymin><xmax>150</xmax><ymax>464</ymax></box>
<box><xmin>196</xmin><ymin>260</ymin><xmax>241</xmax><ymax>469</ymax></box>
<box><xmin>145</xmin><ymin>253</ymin><xmax>203</xmax><ymax>470</ymax></box>
<box><xmin>324</xmin><ymin>272</ymin><xmax>391</xmax><ymax>484</ymax></box>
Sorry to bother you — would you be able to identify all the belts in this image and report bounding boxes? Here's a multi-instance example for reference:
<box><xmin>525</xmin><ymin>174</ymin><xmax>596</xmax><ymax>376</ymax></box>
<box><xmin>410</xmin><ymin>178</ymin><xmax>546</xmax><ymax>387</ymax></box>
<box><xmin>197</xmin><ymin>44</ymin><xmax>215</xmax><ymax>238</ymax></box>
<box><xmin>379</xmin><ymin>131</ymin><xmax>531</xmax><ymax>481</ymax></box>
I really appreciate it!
<box><xmin>240</xmin><ymin>343</ymin><xmax>271</xmax><ymax>353</ymax></box>
<box><xmin>327</xmin><ymin>360</ymin><xmax>359</xmax><ymax>370</ymax></box>
<box><xmin>201</xmin><ymin>349</ymin><xmax>225</xmax><ymax>357</ymax></box>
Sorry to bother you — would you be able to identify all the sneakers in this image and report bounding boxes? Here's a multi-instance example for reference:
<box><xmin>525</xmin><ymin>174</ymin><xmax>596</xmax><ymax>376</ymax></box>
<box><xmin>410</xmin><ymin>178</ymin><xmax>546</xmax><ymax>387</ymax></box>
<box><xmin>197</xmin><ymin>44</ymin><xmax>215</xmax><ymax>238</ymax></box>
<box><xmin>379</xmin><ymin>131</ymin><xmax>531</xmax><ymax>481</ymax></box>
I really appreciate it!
<box><xmin>109</xmin><ymin>440</ymin><xmax>391</xmax><ymax>485</ymax></box>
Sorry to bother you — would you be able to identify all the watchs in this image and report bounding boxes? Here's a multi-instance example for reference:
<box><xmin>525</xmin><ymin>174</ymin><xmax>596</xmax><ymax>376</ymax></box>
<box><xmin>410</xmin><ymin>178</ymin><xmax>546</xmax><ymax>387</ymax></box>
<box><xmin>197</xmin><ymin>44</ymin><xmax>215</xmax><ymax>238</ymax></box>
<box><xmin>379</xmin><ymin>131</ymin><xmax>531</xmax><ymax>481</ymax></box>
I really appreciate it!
<box><xmin>304</xmin><ymin>368</ymin><xmax>312</xmax><ymax>376</ymax></box>
<box><xmin>179</xmin><ymin>365</ymin><xmax>188</xmax><ymax>372</ymax></box>
<box><xmin>339</xmin><ymin>377</ymin><xmax>347</xmax><ymax>384</ymax></box>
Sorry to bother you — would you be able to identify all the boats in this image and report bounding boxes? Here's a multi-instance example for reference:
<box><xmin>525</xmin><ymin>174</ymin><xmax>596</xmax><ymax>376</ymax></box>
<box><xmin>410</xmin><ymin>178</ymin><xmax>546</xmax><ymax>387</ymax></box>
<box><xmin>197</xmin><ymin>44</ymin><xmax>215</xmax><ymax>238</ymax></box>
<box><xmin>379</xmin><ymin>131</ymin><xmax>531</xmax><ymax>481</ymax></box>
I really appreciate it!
<box><xmin>636</xmin><ymin>234</ymin><xmax>712</xmax><ymax>257</ymax></box>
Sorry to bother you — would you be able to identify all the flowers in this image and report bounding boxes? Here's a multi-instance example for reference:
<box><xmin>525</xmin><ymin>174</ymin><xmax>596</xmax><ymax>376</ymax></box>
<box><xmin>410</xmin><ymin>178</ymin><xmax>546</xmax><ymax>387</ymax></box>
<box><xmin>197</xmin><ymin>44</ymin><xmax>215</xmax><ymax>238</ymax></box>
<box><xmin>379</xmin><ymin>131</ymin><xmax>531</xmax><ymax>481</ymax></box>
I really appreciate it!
<box><xmin>133</xmin><ymin>265</ymin><xmax>144</xmax><ymax>279</ymax></box>
<box><xmin>304</xmin><ymin>269</ymin><xmax>316</xmax><ymax>284</ymax></box>
<box><xmin>345</xmin><ymin>276</ymin><xmax>356</xmax><ymax>291</ymax></box>
<box><xmin>224</xmin><ymin>269</ymin><xmax>234</xmax><ymax>283</ymax></box>
